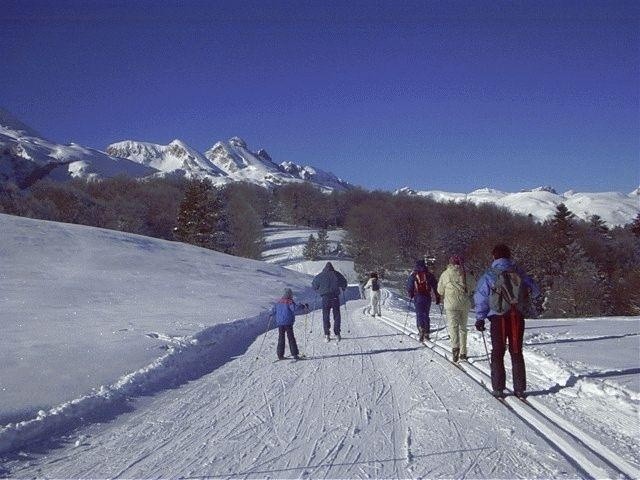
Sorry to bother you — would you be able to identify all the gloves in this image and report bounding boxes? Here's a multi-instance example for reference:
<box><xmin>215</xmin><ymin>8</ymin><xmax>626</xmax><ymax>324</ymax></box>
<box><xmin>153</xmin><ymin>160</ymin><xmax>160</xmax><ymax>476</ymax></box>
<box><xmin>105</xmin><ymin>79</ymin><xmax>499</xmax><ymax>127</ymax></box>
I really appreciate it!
<box><xmin>409</xmin><ymin>292</ymin><xmax>415</xmax><ymax>299</ymax></box>
<box><xmin>475</xmin><ymin>320</ymin><xmax>485</xmax><ymax>331</ymax></box>
<box><xmin>436</xmin><ymin>296</ymin><xmax>441</xmax><ymax>305</ymax></box>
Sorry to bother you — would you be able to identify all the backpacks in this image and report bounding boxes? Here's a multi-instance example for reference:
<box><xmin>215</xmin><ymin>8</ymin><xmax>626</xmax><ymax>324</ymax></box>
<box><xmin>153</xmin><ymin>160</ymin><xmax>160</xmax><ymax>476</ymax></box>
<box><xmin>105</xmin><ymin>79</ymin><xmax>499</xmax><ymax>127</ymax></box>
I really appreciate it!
<box><xmin>372</xmin><ymin>279</ymin><xmax>380</xmax><ymax>291</ymax></box>
<box><xmin>412</xmin><ymin>271</ymin><xmax>429</xmax><ymax>294</ymax></box>
<box><xmin>489</xmin><ymin>265</ymin><xmax>523</xmax><ymax>313</ymax></box>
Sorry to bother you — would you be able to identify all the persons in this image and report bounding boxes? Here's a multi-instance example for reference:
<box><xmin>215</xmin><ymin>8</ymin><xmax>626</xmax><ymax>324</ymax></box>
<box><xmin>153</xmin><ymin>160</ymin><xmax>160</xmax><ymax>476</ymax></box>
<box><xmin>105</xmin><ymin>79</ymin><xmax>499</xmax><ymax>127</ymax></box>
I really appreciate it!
<box><xmin>266</xmin><ymin>286</ymin><xmax>310</xmax><ymax>359</ymax></box>
<box><xmin>437</xmin><ymin>253</ymin><xmax>472</xmax><ymax>362</ymax></box>
<box><xmin>406</xmin><ymin>259</ymin><xmax>442</xmax><ymax>342</ymax></box>
<box><xmin>364</xmin><ymin>273</ymin><xmax>385</xmax><ymax>317</ymax></box>
<box><xmin>474</xmin><ymin>243</ymin><xmax>542</xmax><ymax>398</ymax></box>
<box><xmin>309</xmin><ymin>261</ymin><xmax>347</xmax><ymax>342</ymax></box>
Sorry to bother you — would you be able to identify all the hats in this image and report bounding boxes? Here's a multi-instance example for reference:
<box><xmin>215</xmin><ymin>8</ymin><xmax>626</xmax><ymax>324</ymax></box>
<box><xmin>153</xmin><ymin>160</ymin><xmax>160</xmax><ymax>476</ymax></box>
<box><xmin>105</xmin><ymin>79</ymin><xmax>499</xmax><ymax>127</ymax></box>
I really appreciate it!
<box><xmin>449</xmin><ymin>256</ymin><xmax>463</xmax><ymax>264</ymax></box>
<box><xmin>492</xmin><ymin>244</ymin><xmax>510</xmax><ymax>260</ymax></box>
<box><xmin>283</xmin><ymin>289</ymin><xmax>294</xmax><ymax>299</ymax></box>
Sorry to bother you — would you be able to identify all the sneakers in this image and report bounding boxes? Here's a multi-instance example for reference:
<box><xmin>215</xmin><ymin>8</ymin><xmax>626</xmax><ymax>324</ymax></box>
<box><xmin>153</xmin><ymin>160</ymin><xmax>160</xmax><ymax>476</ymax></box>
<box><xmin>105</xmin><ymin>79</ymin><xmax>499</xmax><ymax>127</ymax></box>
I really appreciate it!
<box><xmin>372</xmin><ymin>314</ymin><xmax>381</xmax><ymax>317</ymax></box>
<box><xmin>491</xmin><ymin>390</ymin><xmax>504</xmax><ymax>397</ymax></box>
<box><xmin>514</xmin><ymin>390</ymin><xmax>524</xmax><ymax>397</ymax></box>
<box><xmin>278</xmin><ymin>353</ymin><xmax>299</xmax><ymax>359</ymax></box>
<box><xmin>325</xmin><ymin>334</ymin><xmax>340</xmax><ymax>342</ymax></box>
<box><xmin>453</xmin><ymin>350</ymin><xmax>466</xmax><ymax>362</ymax></box>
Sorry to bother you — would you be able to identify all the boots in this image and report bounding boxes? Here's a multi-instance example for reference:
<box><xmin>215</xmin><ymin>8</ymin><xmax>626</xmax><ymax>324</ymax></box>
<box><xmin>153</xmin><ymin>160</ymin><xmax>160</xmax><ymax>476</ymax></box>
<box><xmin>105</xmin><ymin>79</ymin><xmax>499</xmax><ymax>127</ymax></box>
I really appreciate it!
<box><xmin>418</xmin><ymin>328</ymin><xmax>429</xmax><ymax>341</ymax></box>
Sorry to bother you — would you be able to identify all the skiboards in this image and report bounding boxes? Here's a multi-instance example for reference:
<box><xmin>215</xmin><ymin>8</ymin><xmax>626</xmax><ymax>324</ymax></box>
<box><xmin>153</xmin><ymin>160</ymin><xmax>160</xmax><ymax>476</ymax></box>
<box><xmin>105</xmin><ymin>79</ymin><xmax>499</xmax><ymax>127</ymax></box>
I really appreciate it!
<box><xmin>481</xmin><ymin>381</ymin><xmax>532</xmax><ymax>411</ymax></box>
<box><xmin>272</xmin><ymin>353</ymin><xmax>306</xmax><ymax>364</ymax></box>
<box><xmin>445</xmin><ymin>353</ymin><xmax>473</xmax><ymax>373</ymax></box>
<box><xmin>417</xmin><ymin>333</ymin><xmax>433</xmax><ymax>347</ymax></box>
